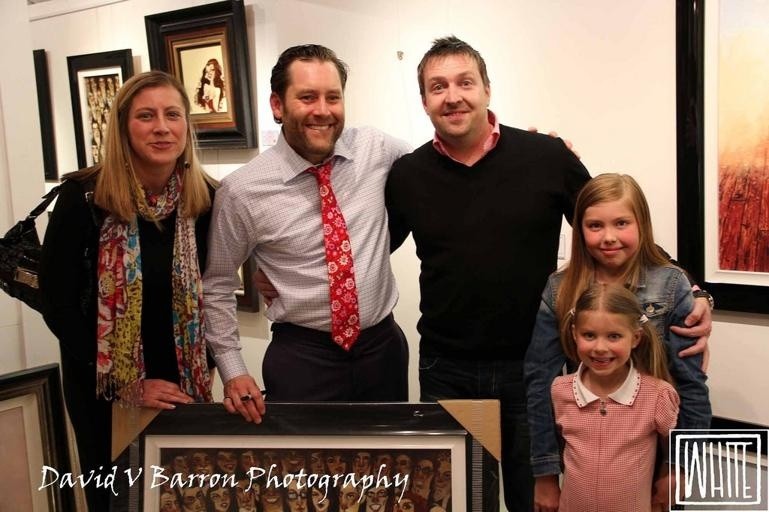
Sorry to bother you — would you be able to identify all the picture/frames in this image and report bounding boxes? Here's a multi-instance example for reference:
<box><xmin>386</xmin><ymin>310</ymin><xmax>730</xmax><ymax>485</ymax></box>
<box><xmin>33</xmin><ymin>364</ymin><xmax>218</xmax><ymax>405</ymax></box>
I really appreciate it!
<box><xmin>1</xmin><ymin>364</ymin><xmax>501</xmax><ymax>512</ymax></box>
<box><xmin>67</xmin><ymin>1</ymin><xmax>258</xmax><ymax>170</ymax></box>
<box><xmin>676</xmin><ymin>0</ymin><xmax>769</xmax><ymax>315</ymax></box>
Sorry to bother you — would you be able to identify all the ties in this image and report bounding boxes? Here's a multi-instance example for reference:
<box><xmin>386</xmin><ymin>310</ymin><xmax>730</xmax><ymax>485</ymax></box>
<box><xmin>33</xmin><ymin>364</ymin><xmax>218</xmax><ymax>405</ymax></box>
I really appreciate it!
<box><xmin>304</xmin><ymin>157</ymin><xmax>361</xmax><ymax>352</ymax></box>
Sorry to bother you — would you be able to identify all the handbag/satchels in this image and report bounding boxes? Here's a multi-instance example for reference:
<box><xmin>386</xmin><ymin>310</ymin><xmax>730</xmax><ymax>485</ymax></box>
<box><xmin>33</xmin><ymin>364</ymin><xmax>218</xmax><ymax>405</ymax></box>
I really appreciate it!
<box><xmin>0</xmin><ymin>218</ymin><xmax>44</xmax><ymax>314</ymax></box>
